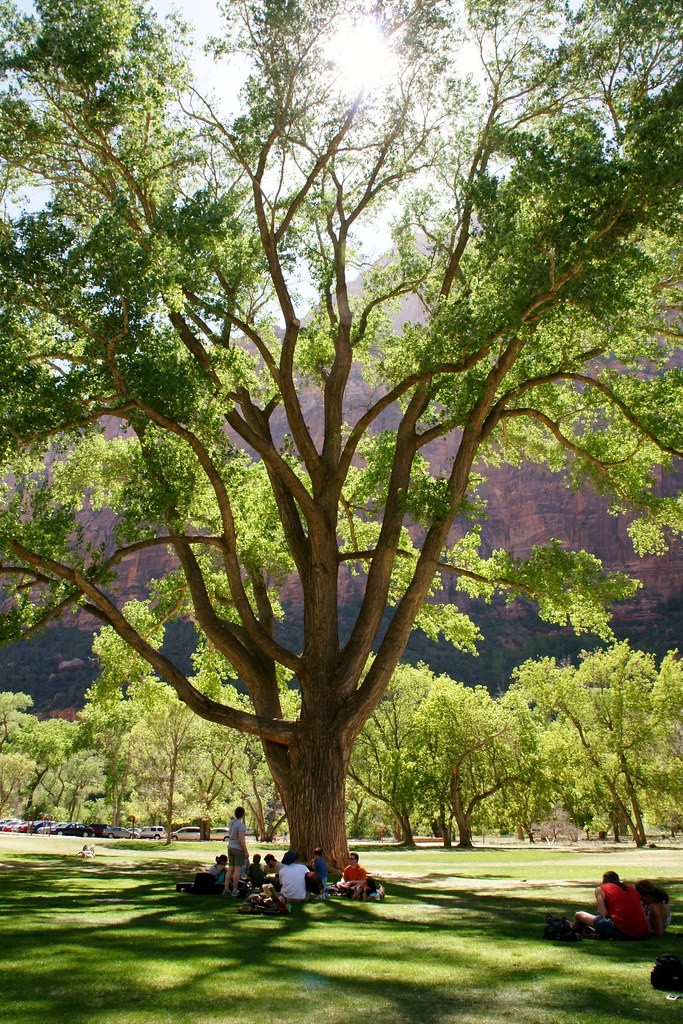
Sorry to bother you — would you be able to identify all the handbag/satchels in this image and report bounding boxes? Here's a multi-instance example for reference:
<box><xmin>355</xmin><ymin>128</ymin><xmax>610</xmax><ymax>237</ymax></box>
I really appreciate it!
<box><xmin>543</xmin><ymin>915</ymin><xmax>582</xmax><ymax>942</ymax></box>
<box><xmin>651</xmin><ymin>955</ymin><xmax>683</xmax><ymax>991</ymax></box>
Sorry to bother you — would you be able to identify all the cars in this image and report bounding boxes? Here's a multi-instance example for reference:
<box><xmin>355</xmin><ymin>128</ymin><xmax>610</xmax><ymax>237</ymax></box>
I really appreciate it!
<box><xmin>0</xmin><ymin>818</ymin><xmax>67</xmax><ymax>834</ymax></box>
<box><xmin>172</xmin><ymin>827</ymin><xmax>202</xmax><ymax>841</ymax></box>
<box><xmin>88</xmin><ymin>823</ymin><xmax>110</xmax><ymax>838</ymax></box>
<box><xmin>50</xmin><ymin>823</ymin><xmax>94</xmax><ymax>838</ymax></box>
<box><xmin>210</xmin><ymin>828</ymin><xmax>229</xmax><ymax>842</ymax></box>
<box><xmin>129</xmin><ymin>828</ymin><xmax>141</xmax><ymax>834</ymax></box>
<box><xmin>102</xmin><ymin>827</ymin><xmax>138</xmax><ymax>839</ymax></box>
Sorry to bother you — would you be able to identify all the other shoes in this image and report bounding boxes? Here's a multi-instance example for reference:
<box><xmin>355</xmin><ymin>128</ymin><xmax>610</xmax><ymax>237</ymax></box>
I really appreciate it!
<box><xmin>231</xmin><ymin>890</ymin><xmax>240</xmax><ymax>896</ymax></box>
<box><xmin>223</xmin><ymin>889</ymin><xmax>231</xmax><ymax>895</ymax></box>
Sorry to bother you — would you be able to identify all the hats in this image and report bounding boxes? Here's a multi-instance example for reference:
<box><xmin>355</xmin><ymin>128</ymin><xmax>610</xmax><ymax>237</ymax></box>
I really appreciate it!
<box><xmin>282</xmin><ymin>851</ymin><xmax>295</xmax><ymax>865</ymax></box>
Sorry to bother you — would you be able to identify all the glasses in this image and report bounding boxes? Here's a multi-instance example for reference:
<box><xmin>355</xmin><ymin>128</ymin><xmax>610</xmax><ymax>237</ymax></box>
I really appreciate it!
<box><xmin>348</xmin><ymin>858</ymin><xmax>353</xmax><ymax>860</ymax></box>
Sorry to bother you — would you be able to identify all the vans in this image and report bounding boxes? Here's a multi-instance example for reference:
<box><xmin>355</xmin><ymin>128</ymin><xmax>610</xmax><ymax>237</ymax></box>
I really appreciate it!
<box><xmin>138</xmin><ymin>826</ymin><xmax>165</xmax><ymax>839</ymax></box>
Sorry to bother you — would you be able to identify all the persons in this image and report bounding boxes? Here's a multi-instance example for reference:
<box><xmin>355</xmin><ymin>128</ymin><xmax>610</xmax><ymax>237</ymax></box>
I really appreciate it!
<box><xmin>76</xmin><ymin>845</ymin><xmax>95</xmax><ymax>858</ymax></box>
<box><xmin>634</xmin><ymin>879</ymin><xmax>672</xmax><ymax>936</ymax></box>
<box><xmin>566</xmin><ymin>871</ymin><xmax>649</xmax><ymax>941</ymax></box>
<box><xmin>208</xmin><ymin>846</ymin><xmax>386</xmax><ymax>911</ymax></box>
<box><xmin>222</xmin><ymin>807</ymin><xmax>250</xmax><ymax>897</ymax></box>
